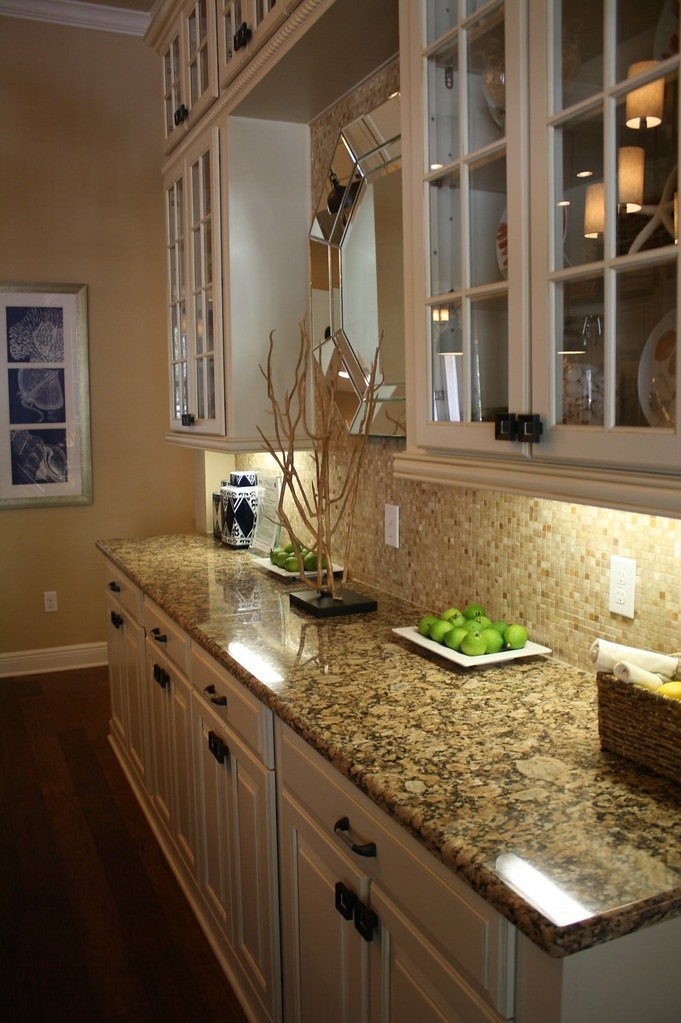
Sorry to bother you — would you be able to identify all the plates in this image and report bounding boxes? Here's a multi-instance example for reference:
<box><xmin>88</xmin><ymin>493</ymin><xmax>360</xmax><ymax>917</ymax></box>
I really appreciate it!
<box><xmin>653</xmin><ymin>0</ymin><xmax>681</xmax><ymax>82</ymax></box>
<box><xmin>497</xmin><ymin>197</ymin><xmax>568</xmax><ymax>280</ymax></box>
<box><xmin>392</xmin><ymin>626</ymin><xmax>552</xmax><ymax>668</ymax></box>
<box><xmin>638</xmin><ymin>308</ymin><xmax>676</xmax><ymax>429</ymax></box>
<box><xmin>252</xmin><ymin>558</ymin><xmax>345</xmax><ymax>578</ymax></box>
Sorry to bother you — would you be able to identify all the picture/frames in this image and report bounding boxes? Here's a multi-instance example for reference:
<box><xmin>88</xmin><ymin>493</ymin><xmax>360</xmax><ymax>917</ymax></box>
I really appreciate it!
<box><xmin>0</xmin><ymin>282</ymin><xmax>94</xmax><ymax>511</ymax></box>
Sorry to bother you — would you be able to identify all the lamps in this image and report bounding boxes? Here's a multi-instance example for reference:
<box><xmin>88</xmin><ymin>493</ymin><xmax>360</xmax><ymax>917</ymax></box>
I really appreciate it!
<box><xmin>626</xmin><ymin>61</ymin><xmax>665</xmax><ymax>129</ymax></box>
<box><xmin>616</xmin><ymin>147</ymin><xmax>644</xmax><ymax>215</ymax></box>
<box><xmin>583</xmin><ymin>182</ymin><xmax>606</xmax><ymax>238</ymax></box>
<box><xmin>432</xmin><ymin>308</ymin><xmax>450</xmax><ymax>322</ymax></box>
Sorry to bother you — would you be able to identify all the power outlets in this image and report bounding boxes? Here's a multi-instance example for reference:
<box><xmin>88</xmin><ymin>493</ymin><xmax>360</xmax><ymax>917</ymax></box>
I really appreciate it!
<box><xmin>44</xmin><ymin>591</ymin><xmax>58</xmax><ymax>613</ymax></box>
<box><xmin>384</xmin><ymin>503</ymin><xmax>399</xmax><ymax>549</ymax></box>
<box><xmin>609</xmin><ymin>554</ymin><xmax>636</xmax><ymax>620</ymax></box>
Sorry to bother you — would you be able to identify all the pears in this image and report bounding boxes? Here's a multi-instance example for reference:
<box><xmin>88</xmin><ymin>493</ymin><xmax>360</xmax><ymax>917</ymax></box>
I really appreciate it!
<box><xmin>415</xmin><ymin>605</ymin><xmax>527</xmax><ymax>656</ymax></box>
<box><xmin>269</xmin><ymin>540</ymin><xmax>327</xmax><ymax>572</ymax></box>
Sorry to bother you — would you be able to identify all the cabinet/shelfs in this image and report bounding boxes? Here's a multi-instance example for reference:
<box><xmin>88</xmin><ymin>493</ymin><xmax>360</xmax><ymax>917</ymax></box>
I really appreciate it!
<box><xmin>98</xmin><ymin>554</ymin><xmax>681</xmax><ymax>1023</ymax></box>
<box><xmin>161</xmin><ymin>114</ymin><xmax>324</xmax><ymax>454</ymax></box>
<box><xmin>392</xmin><ymin>0</ymin><xmax>681</xmax><ymax>521</ymax></box>
<box><xmin>141</xmin><ymin>0</ymin><xmax>398</xmax><ymax>174</ymax></box>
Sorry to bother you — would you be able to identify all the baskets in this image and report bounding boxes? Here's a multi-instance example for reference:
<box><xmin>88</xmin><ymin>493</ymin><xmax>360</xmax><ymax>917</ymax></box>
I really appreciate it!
<box><xmin>597</xmin><ymin>652</ymin><xmax>681</xmax><ymax>783</ymax></box>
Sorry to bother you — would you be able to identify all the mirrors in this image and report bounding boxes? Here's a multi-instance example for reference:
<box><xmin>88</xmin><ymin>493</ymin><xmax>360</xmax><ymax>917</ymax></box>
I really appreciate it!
<box><xmin>310</xmin><ymin>87</ymin><xmax>408</xmax><ymax>439</ymax></box>
